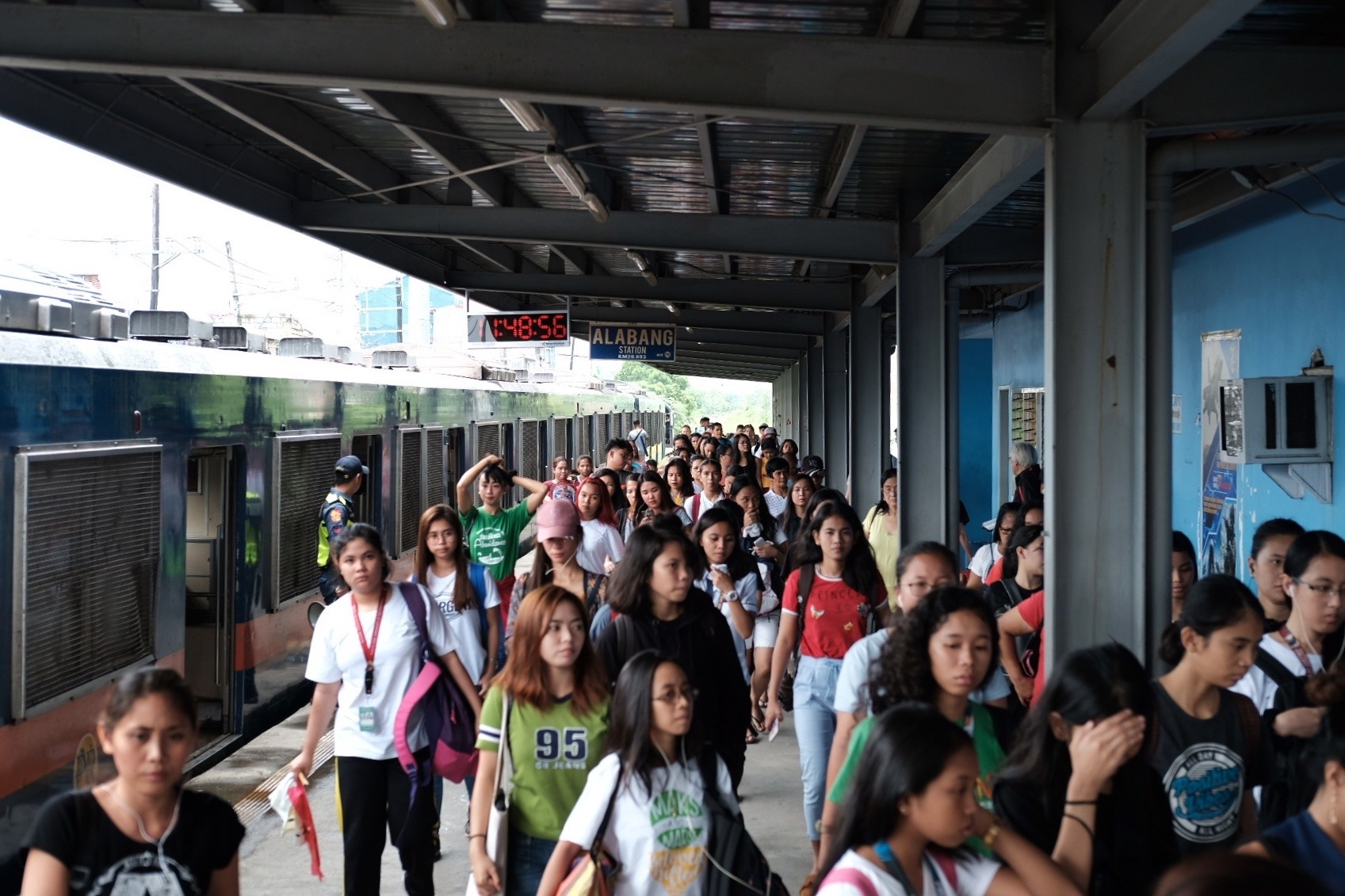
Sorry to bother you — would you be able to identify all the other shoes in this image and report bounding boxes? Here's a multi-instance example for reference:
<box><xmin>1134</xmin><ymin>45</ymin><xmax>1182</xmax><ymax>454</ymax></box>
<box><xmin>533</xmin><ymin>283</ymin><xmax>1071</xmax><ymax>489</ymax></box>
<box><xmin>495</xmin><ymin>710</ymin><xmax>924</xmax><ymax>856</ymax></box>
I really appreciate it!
<box><xmin>799</xmin><ymin>874</ymin><xmax>826</xmax><ymax>896</ymax></box>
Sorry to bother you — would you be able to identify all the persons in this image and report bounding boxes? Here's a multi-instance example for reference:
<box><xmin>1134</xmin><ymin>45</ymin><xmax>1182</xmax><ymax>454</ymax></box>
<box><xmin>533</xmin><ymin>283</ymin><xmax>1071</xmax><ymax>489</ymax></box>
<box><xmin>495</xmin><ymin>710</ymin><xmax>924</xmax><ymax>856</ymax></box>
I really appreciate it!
<box><xmin>291</xmin><ymin>417</ymin><xmax>1345</xmax><ymax>896</ymax></box>
<box><xmin>18</xmin><ymin>668</ymin><xmax>247</xmax><ymax>896</ymax></box>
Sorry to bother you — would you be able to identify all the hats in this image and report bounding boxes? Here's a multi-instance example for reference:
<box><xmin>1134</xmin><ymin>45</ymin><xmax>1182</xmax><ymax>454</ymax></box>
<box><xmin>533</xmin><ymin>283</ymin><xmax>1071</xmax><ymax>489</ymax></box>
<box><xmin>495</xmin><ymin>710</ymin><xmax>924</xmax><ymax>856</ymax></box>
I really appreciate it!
<box><xmin>798</xmin><ymin>455</ymin><xmax>827</xmax><ymax>477</ymax></box>
<box><xmin>334</xmin><ymin>455</ymin><xmax>369</xmax><ymax>479</ymax></box>
<box><xmin>536</xmin><ymin>497</ymin><xmax>581</xmax><ymax>543</ymax></box>
<box><xmin>759</xmin><ymin>423</ymin><xmax>768</xmax><ymax>429</ymax></box>
<box><xmin>764</xmin><ymin>427</ymin><xmax>780</xmax><ymax>437</ymax></box>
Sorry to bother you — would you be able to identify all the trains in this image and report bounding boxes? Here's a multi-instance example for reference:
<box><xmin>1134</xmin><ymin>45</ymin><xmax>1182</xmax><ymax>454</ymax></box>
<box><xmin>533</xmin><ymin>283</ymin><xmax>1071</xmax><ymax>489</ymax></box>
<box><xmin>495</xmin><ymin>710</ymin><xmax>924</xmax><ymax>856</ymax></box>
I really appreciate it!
<box><xmin>0</xmin><ymin>331</ymin><xmax>672</xmax><ymax>806</ymax></box>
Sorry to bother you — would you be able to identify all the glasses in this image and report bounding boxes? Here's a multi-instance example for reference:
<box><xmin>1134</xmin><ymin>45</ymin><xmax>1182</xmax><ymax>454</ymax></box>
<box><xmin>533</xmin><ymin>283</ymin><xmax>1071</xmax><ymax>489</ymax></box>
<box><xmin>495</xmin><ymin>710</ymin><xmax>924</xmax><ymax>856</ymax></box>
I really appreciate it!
<box><xmin>738</xmin><ymin>441</ymin><xmax>748</xmax><ymax>444</ymax></box>
<box><xmin>900</xmin><ymin>581</ymin><xmax>950</xmax><ymax>596</ymax></box>
<box><xmin>999</xmin><ymin>525</ymin><xmax>1013</xmax><ymax>535</ymax></box>
<box><xmin>882</xmin><ymin>486</ymin><xmax>897</xmax><ymax>493</ymax></box>
<box><xmin>1293</xmin><ymin>577</ymin><xmax>1345</xmax><ymax>596</ymax></box>
<box><xmin>725</xmin><ymin>453</ymin><xmax>734</xmax><ymax>457</ymax></box>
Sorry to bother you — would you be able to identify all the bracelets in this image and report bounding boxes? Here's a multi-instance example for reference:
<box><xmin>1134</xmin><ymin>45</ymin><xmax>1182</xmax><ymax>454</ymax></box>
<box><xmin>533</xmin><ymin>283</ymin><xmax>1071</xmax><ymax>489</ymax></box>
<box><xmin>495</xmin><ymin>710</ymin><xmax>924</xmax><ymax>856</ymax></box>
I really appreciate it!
<box><xmin>488</xmin><ymin>657</ymin><xmax>500</xmax><ymax>670</ymax></box>
<box><xmin>1061</xmin><ymin>813</ymin><xmax>1096</xmax><ymax>841</ymax></box>
<box><xmin>468</xmin><ymin>833</ymin><xmax>488</xmax><ymax>841</ymax></box>
<box><xmin>983</xmin><ymin>816</ymin><xmax>1004</xmax><ymax>846</ymax></box>
<box><xmin>1064</xmin><ymin>799</ymin><xmax>1098</xmax><ymax>806</ymax></box>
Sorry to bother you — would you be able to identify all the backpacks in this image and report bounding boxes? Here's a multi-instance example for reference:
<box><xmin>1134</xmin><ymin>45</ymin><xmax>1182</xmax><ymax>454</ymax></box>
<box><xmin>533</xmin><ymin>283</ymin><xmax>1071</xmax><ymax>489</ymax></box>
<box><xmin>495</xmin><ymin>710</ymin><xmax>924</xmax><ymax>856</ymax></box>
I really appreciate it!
<box><xmin>695</xmin><ymin>749</ymin><xmax>791</xmax><ymax>896</ymax></box>
<box><xmin>1253</xmin><ymin>645</ymin><xmax>1345</xmax><ymax>835</ymax></box>
<box><xmin>394</xmin><ymin>581</ymin><xmax>484</xmax><ymax>785</ymax></box>
<box><xmin>994</xmin><ymin>578</ymin><xmax>1044</xmax><ymax>716</ymax></box>
<box><xmin>412</xmin><ymin>562</ymin><xmax>505</xmax><ymax>664</ymax></box>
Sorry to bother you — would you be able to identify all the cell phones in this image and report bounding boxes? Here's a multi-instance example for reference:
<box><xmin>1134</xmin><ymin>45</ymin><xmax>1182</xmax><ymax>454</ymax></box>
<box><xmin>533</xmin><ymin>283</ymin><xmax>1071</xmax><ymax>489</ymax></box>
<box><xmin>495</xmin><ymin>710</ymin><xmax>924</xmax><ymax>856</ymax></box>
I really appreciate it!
<box><xmin>754</xmin><ymin>538</ymin><xmax>765</xmax><ymax>547</ymax></box>
<box><xmin>641</xmin><ymin>509</ymin><xmax>655</xmax><ymax>521</ymax></box>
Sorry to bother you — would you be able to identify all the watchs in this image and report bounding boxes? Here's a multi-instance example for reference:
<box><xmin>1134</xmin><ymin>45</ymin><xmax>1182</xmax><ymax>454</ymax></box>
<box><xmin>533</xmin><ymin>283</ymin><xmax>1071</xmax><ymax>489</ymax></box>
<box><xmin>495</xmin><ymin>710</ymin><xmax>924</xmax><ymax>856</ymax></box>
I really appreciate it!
<box><xmin>721</xmin><ymin>589</ymin><xmax>740</xmax><ymax>603</ymax></box>
<box><xmin>815</xmin><ymin>819</ymin><xmax>838</xmax><ymax>834</ymax></box>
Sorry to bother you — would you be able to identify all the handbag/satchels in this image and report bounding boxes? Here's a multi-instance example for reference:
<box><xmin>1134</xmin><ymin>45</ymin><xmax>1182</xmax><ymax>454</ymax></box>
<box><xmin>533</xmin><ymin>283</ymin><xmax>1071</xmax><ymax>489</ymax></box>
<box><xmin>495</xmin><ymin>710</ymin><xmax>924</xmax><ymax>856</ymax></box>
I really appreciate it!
<box><xmin>462</xmin><ymin>510</ymin><xmax>477</xmax><ymax>560</ymax></box>
<box><xmin>556</xmin><ymin>847</ymin><xmax>624</xmax><ymax>896</ymax></box>
<box><xmin>464</xmin><ymin>684</ymin><xmax>510</xmax><ymax>896</ymax></box>
<box><xmin>777</xmin><ymin>672</ymin><xmax>795</xmax><ymax>712</ymax></box>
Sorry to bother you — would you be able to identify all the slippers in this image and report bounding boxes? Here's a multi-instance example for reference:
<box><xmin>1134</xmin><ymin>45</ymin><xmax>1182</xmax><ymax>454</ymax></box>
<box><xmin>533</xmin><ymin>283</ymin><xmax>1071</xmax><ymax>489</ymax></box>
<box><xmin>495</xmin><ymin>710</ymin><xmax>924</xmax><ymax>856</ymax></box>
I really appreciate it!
<box><xmin>758</xmin><ymin>696</ymin><xmax>769</xmax><ymax>708</ymax></box>
<box><xmin>745</xmin><ymin>708</ymin><xmax>765</xmax><ymax>744</ymax></box>
